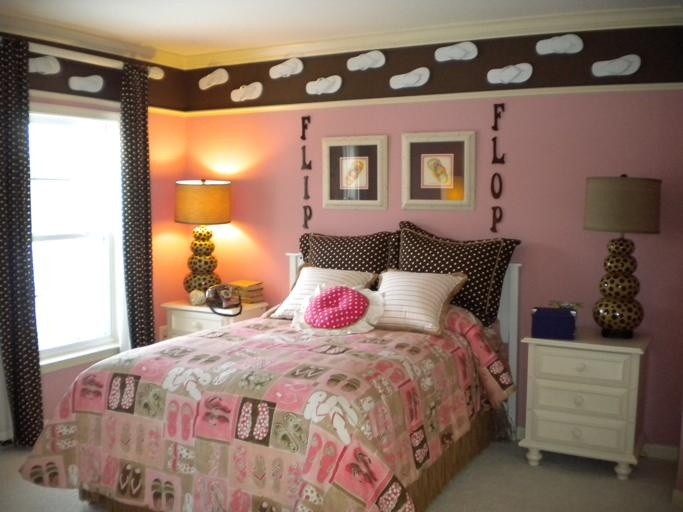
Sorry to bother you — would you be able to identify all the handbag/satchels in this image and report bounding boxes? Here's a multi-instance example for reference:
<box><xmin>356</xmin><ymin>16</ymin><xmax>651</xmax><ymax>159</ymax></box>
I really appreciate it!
<box><xmin>204</xmin><ymin>283</ymin><xmax>243</xmax><ymax>317</ymax></box>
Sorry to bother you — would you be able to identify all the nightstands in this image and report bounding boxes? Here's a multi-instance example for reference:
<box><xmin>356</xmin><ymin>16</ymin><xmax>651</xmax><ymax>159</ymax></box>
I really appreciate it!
<box><xmin>517</xmin><ymin>332</ymin><xmax>653</xmax><ymax>481</ymax></box>
<box><xmin>161</xmin><ymin>301</ymin><xmax>269</xmax><ymax>340</ymax></box>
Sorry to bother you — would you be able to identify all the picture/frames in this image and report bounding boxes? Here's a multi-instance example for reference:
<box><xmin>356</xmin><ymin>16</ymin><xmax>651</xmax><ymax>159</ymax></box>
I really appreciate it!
<box><xmin>319</xmin><ymin>132</ymin><xmax>389</xmax><ymax>213</ymax></box>
<box><xmin>399</xmin><ymin>131</ymin><xmax>476</xmax><ymax>210</ymax></box>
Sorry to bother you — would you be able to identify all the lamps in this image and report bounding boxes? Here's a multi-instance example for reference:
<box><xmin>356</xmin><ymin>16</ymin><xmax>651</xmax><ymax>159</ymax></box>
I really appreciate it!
<box><xmin>172</xmin><ymin>177</ymin><xmax>233</xmax><ymax>295</ymax></box>
<box><xmin>578</xmin><ymin>173</ymin><xmax>664</xmax><ymax>339</ymax></box>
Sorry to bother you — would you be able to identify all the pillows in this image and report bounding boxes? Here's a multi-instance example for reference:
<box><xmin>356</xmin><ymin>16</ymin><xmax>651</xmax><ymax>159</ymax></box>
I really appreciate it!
<box><xmin>264</xmin><ymin>220</ymin><xmax>522</xmax><ymax>338</ymax></box>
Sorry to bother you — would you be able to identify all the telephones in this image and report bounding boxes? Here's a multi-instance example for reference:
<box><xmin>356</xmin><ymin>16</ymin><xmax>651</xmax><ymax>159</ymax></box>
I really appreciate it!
<box><xmin>206</xmin><ymin>283</ymin><xmax>240</xmax><ymax>309</ymax></box>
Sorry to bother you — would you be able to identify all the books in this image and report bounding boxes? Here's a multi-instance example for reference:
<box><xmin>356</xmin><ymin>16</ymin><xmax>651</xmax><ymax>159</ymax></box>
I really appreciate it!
<box><xmin>227</xmin><ymin>279</ymin><xmax>264</xmax><ymax>303</ymax></box>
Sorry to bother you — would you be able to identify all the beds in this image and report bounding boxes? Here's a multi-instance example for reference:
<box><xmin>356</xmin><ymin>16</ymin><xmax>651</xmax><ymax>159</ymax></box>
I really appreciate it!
<box><xmin>20</xmin><ymin>250</ymin><xmax>523</xmax><ymax>511</ymax></box>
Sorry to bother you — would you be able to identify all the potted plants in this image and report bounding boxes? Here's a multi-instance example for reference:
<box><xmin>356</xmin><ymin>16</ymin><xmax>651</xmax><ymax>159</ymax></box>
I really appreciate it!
<box><xmin>529</xmin><ymin>297</ymin><xmax>585</xmax><ymax>340</ymax></box>
